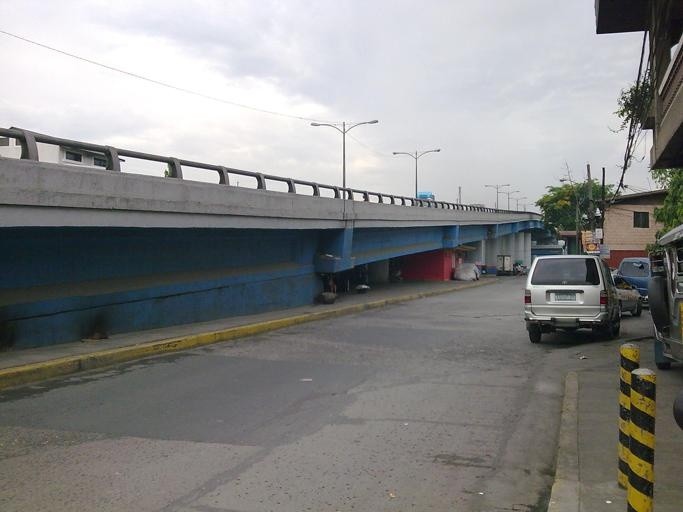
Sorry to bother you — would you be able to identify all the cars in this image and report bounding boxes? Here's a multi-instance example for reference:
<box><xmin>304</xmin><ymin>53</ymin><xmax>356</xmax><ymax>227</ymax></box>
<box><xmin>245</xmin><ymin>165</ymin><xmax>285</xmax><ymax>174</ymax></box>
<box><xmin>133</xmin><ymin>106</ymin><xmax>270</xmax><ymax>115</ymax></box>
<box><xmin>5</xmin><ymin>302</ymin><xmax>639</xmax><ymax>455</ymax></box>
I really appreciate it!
<box><xmin>610</xmin><ymin>271</ymin><xmax>643</xmax><ymax>317</ymax></box>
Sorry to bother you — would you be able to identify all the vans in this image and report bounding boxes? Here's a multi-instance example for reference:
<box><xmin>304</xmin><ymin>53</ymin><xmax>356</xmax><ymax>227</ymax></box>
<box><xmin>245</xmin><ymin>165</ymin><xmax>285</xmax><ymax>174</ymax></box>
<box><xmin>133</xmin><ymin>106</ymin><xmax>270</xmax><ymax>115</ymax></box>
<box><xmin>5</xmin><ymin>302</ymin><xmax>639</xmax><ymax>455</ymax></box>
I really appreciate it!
<box><xmin>522</xmin><ymin>253</ymin><xmax>620</xmax><ymax>341</ymax></box>
<box><xmin>619</xmin><ymin>257</ymin><xmax>650</xmax><ymax>308</ymax></box>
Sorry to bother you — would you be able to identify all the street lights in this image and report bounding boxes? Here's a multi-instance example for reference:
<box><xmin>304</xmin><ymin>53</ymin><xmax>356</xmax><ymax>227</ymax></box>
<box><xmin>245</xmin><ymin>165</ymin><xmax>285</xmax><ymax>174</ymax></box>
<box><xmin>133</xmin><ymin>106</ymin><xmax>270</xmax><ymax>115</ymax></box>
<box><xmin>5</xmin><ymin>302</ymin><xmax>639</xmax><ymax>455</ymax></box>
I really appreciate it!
<box><xmin>484</xmin><ymin>183</ymin><xmax>535</xmax><ymax>212</ymax></box>
<box><xmin>310</xmin><ymin>119</ymin><xmax>378</xmax><ymax>200</ymax></box>
<box><xmin>393</xmin><ymin>148</ymin><xmax>441</xmax><ymax>206</ymax></box>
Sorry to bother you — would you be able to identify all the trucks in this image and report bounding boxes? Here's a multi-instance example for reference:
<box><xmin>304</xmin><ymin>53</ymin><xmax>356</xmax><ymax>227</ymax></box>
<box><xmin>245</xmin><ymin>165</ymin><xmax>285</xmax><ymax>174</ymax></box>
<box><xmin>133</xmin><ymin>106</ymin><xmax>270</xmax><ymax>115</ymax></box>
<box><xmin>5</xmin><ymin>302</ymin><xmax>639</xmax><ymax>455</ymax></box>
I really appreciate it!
<box><xmin>496</xmin><ymin>254</ymin><xmax>513</xmax><ymax>276</ymax></box>
<box><xmin>417</xmin><ymin>191</ymin><xmax>435</xmax><ymax>207</ymax></box>
